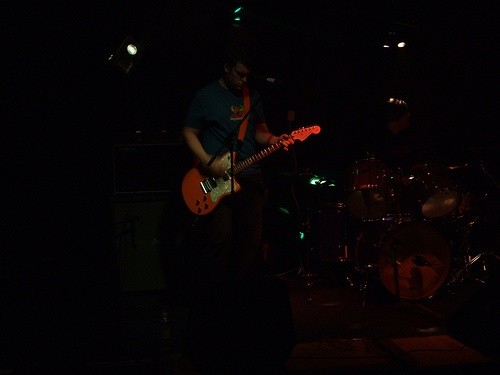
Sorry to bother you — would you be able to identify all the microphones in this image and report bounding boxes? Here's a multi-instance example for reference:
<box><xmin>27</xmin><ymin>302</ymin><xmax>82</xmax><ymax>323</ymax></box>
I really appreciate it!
<box><xmin>264</xmin><ymin>77</ymin><xmax>283</xmax><ymax>85</ymax></box>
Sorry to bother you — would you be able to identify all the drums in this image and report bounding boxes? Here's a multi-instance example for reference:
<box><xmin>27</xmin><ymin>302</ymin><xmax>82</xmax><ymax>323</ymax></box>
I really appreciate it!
<box><xmin>414</xmin><ymin>165</ymin><xmax>462</xmax><ymax>219</ymax></box>
<box><xmin>346</xmin><ymin>158</ymin><xmax>398</xmax><ymax>223</ymax></box>
<box><xmin>356</xmin><ymin>221</ymin><xmax>454</xmax><ymax>299</ymax></box>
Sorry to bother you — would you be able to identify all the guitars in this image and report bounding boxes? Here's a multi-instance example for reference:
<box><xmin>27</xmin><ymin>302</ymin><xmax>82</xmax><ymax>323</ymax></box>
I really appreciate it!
<box><xmin>181</xmin><ymin>123</ymin><xmax>322</xmax><ymax>216</ymax></box>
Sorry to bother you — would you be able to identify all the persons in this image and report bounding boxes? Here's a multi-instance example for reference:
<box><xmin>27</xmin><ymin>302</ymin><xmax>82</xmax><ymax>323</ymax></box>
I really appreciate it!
<box><xmin>373</xmin><ymin>98</ymin><xmax>421</xmax><ymax>183</ymax></box>
<box><xmin>182</xmin><ymin>49</ymin><xmax>296</xmax><ymax>283</ymax></box>
